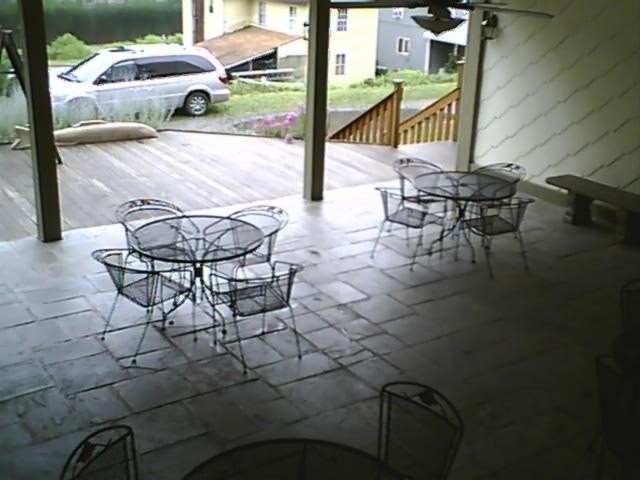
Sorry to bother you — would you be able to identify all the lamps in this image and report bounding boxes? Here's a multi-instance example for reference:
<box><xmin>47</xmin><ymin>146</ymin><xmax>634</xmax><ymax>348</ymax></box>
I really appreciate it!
<box><xmin>478</xmin><ymin>14</ymin><xmax>497</xmax><ymax>40</ymax></box>
<box><xmin>410</xmin><ymin>8</ymin><xmax>464</xmax><ymax>36</ymax></box>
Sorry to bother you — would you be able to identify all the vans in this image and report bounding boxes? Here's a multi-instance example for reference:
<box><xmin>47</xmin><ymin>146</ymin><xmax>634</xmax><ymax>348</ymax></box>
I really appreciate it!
<box><xmin>49</xmin><ymin>39</ymin><xmax>232</xmax><ymax>129</ymax></box>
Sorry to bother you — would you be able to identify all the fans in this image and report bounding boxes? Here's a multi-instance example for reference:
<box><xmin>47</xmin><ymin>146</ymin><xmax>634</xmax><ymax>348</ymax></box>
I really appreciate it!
<box><xmin>327</xmin><ymin>1</ymin><xmax>557</xmax><ymax>36</ymax></box>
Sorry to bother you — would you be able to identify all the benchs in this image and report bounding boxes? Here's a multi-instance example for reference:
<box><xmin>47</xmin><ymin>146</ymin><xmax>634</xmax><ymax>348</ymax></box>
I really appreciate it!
<box><xmin>546</xmin><ymin>174</ymin><xmax>640</xmax><ymax>243</ymax></box>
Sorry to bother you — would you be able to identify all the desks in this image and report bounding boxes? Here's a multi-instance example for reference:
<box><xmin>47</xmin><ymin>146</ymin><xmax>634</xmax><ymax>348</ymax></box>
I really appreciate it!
<box><xmin>182</xmin><ymin>437</ymin><xmax>405</xmax><ymax>480</ymax></box>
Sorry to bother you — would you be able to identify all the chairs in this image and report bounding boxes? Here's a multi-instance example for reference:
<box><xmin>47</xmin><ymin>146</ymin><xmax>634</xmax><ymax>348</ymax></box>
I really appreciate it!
<box><xmin>89</xmin><ymin>199</ymin><xmax>304</xmax><ymax>378</ymax></box>
<box><xmin>378</xmin><ymin>380</ymin><xmax>466</xmax><ymax>477</ymax></box>
<box><xmin>369</xmin><ymin>156</ymin><xmax>535</xmax><ymax>278</ymax></box>
<box><xmin>63</xmin><ymin>424</ymin><xmax>142</xmax><ymax>478</ymax></box>
